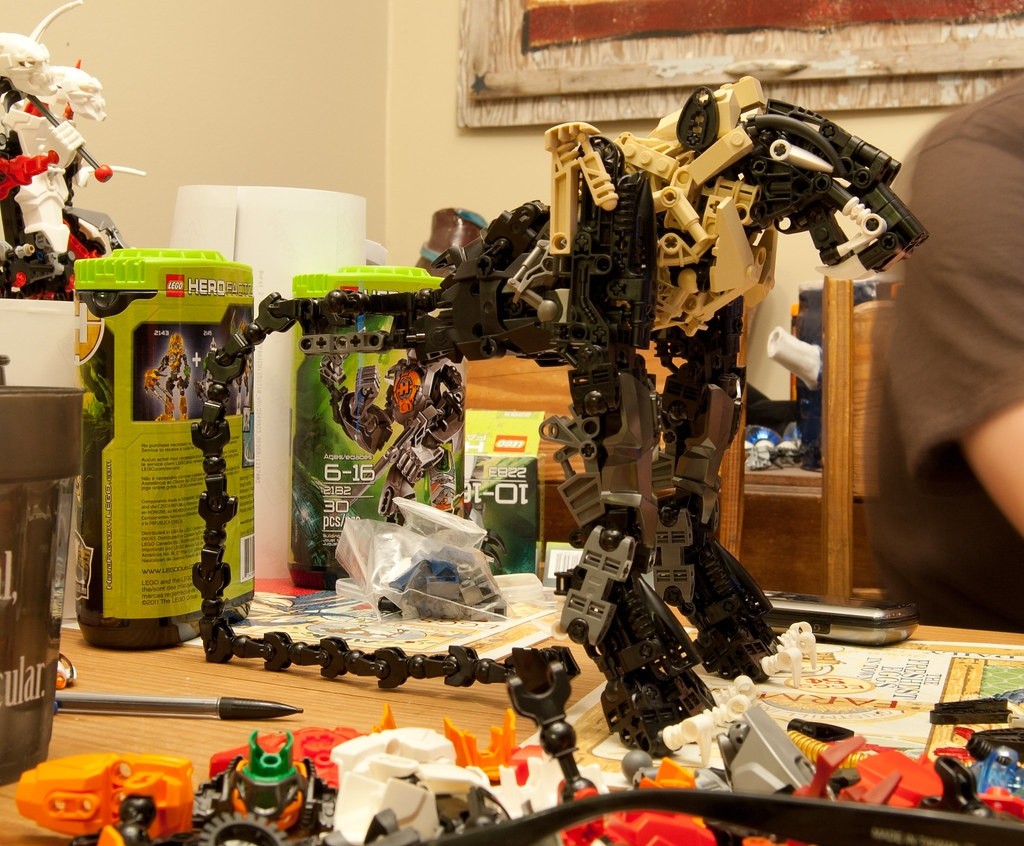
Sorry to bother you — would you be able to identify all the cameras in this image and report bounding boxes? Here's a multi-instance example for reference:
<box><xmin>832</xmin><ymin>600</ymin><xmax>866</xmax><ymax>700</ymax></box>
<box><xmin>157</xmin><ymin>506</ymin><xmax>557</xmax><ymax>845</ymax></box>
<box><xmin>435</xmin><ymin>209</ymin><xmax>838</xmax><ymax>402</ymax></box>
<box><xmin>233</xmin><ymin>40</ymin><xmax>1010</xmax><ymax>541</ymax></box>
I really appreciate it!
<box><xmin>760</xmin><ymin>589</ymin><xmax>919</xmax><ymax>647</ymax></box>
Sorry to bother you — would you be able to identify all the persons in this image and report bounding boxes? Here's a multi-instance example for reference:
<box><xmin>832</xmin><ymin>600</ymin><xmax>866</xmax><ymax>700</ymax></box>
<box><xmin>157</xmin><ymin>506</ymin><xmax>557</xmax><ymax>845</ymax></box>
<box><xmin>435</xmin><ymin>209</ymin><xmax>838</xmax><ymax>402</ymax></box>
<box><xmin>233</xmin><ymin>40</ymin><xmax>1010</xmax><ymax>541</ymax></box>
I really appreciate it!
<box><xmin>862</xmin><ymin>77</ymin><xmax>1024</xmax><ymax>634</ymax></box>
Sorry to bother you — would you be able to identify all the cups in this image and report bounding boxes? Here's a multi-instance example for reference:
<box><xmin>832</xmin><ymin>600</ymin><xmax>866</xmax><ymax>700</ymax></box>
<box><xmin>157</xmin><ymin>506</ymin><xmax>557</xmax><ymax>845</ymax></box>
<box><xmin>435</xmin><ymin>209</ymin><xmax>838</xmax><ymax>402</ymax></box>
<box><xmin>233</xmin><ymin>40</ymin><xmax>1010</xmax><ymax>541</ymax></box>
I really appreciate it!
<box><xmin>0</xmin><ymin>389</ymin><xmax>87</xmax><ymax>787</ymax></box>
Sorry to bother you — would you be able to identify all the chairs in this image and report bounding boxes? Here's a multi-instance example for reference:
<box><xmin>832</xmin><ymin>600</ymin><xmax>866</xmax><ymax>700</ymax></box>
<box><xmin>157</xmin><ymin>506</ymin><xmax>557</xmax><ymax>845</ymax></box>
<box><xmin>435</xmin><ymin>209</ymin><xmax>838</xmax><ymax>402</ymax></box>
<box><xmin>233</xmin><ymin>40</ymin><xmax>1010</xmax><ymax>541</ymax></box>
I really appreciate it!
<box><xmin>821</xmin><ymin>274</ymin><xmax>896</xmax><ymax>600</ymax></box>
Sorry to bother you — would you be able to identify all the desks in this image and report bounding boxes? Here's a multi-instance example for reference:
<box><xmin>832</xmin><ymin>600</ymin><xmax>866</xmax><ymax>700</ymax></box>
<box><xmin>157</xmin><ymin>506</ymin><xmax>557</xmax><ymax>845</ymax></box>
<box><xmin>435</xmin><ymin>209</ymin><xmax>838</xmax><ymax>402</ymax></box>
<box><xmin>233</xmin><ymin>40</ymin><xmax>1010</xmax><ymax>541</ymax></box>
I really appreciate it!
<box><xmin>0</xmin><ymin>618</ymin><xmax>1024</xmax><ymax>797</ymax></box>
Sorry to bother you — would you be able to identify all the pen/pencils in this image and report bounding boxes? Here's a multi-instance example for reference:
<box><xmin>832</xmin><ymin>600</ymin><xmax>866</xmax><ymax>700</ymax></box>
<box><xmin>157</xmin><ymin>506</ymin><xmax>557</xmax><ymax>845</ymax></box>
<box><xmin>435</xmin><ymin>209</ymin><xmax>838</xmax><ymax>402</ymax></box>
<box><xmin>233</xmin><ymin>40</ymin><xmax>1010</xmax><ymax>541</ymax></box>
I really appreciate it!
<box><xmin>54</xmin><ymin>692</ymin><xmax>309</xmax><ymax>723</ymax></box>
